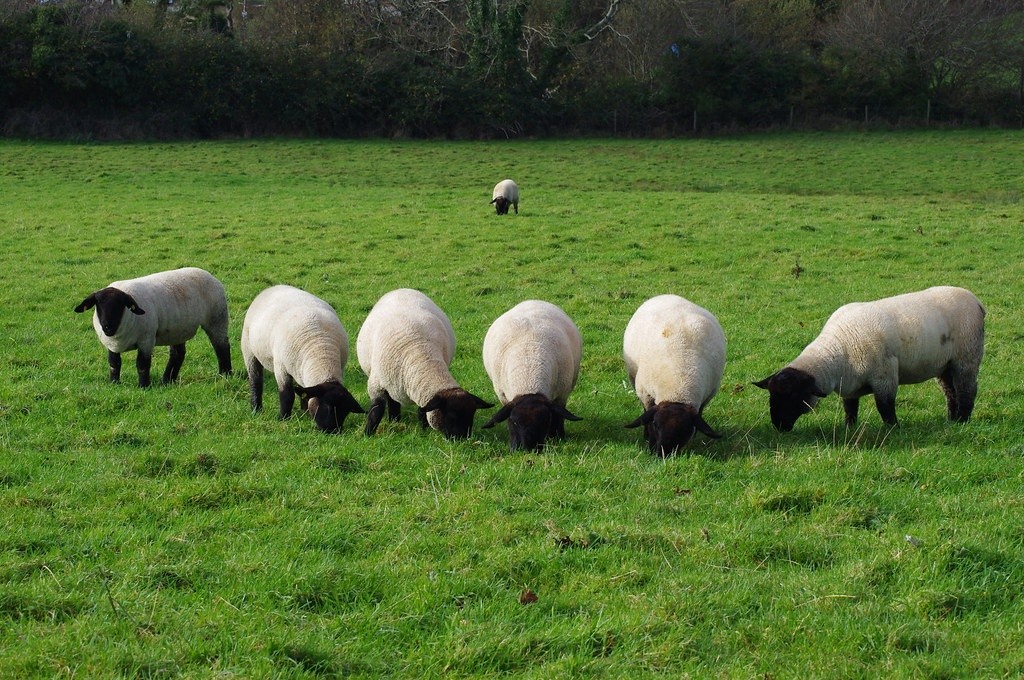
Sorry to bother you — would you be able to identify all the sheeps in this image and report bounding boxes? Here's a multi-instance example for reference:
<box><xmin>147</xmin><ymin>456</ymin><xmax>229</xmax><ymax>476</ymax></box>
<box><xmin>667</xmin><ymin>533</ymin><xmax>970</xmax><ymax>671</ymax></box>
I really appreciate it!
<box><xmin>72</xmin><ymin>265</ymin><xmax>233</xmax><ymax>390</ymax></box>
<box><xmin>490</xmin><ymin>179</ymin><xmax>519</xmax><ymax>215</ymax></box>
<box><xmin>239</xmin><ymin>283</ymin><xmax>370</xmax><ymax>435</ymax></box>
<box><xmin>745</xmin><ymin>285</ymin><xmax>987</xmax><ymax>439</ymax></box>
<box><xmin>355</xmin><ymin>288</ymin><xmax>497</xmax><ymax>444</ymax></box>
<box><xmin>480</xmin><ymin>298</ymin><xmax>582</xmax><ymax>455</ymax></box>
<box><xmin>621</xmin><ymin>294</ymin><xmax>729</xmax><ymax>459</ymax></box>
